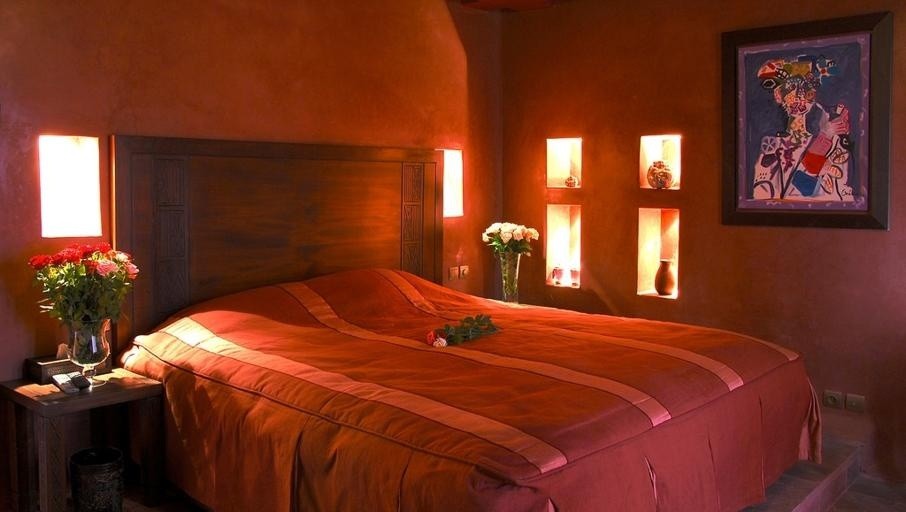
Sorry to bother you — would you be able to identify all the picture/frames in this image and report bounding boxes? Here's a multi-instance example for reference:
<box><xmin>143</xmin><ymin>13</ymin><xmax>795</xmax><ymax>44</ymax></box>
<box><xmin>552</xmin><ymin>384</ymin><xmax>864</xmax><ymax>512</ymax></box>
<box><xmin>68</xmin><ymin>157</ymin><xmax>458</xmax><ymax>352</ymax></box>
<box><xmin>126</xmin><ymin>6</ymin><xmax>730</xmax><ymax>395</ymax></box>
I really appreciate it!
<box><xmin>722</xmin><ymin>11</ymin><xmax>893</xmax><ymax>229</ymax></box>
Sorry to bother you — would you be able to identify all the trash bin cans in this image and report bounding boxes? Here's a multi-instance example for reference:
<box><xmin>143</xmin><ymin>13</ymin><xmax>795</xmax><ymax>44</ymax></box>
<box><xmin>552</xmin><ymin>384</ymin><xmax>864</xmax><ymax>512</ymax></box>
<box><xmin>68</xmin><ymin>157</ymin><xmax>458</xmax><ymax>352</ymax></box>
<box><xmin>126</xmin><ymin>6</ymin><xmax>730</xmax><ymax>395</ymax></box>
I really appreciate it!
<box><xmin>69</xmin><ymin>447</ymin><xmax>124</xmax><ymax>512</ymax></box>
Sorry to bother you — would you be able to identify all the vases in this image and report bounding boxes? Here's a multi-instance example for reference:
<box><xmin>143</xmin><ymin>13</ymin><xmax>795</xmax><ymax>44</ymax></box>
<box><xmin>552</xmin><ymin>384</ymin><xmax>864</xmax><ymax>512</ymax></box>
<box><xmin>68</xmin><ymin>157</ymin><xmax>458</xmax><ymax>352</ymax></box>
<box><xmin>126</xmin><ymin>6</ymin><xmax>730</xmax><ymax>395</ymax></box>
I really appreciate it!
<box><xmin>647</xmin><ymin>161</ymin><xmax>673</xmax><ymax>190</ymax></box>
<box><xmin>66</xmin><ymin>320</ymin><xmax>110</xmax><ymax>388</ymax></box>
<box><xmin>110</xmin><ymin>131</ymin><xmax>823</xmax><ymax>512</ymax></box>
<box><xmin>500</xmin><ymin>252</ymin><xmax>521</xmax><ymax>302</ymax></box>
<box><xmin>655</xmin><ymin>259</ymin><xmax>674</xmax><ymax>295</ymax></box>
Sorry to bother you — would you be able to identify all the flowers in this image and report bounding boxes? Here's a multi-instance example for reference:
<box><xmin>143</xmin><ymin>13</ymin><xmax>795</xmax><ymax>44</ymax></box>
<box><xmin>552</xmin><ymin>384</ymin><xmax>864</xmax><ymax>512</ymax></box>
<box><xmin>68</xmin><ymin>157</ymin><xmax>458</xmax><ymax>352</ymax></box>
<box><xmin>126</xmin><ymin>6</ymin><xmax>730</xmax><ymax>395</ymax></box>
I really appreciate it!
<box><xmin>426</xmin><ymin>314</ymin><xmax>500</xmax><ymax>348</ymax></box>
<box><xmin>27</xmin><ymin>243</ymin><xmax>139</xmax><ymax>325</ymax></box>
<box><xmin>481</xmin><ymin>221</ymin><xmax>540</xmax><ymax>255</ymax></box>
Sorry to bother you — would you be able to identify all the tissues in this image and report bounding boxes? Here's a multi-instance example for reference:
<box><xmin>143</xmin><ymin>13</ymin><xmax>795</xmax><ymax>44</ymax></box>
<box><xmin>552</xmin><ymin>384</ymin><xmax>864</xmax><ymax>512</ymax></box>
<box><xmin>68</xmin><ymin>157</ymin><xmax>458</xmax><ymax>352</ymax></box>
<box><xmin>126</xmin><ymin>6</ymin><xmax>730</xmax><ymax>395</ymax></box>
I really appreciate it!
<box><xmin>23</xmin><ymin>343</ymin><xmax>84</xmax><ymax>385</ymax></box>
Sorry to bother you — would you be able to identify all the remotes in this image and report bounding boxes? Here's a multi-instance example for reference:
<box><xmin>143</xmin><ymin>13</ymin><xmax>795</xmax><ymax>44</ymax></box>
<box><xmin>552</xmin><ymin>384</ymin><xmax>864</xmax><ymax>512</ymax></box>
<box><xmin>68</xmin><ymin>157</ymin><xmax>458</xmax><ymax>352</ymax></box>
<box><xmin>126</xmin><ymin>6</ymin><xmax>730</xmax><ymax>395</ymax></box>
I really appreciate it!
<box><xmin>67</xmin><ymin>371</ymin><xmax>90</xmax><ymax>390</ymax></box>
<box><xmin>51</xmin><ymin>374</ymin><xmax>80</xmax><ymax>394</ymax></box>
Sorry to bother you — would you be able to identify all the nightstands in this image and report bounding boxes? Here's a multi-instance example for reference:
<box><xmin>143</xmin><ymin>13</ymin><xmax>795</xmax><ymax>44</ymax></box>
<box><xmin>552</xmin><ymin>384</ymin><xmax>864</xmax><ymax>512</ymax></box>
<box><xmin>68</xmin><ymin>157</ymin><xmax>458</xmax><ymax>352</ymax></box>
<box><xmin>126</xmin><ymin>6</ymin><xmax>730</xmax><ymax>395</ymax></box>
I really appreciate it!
<box><xmin>0</xmin><ymin>366</ymin><xmax>164</xmax><ymax>511</ymax></box>
